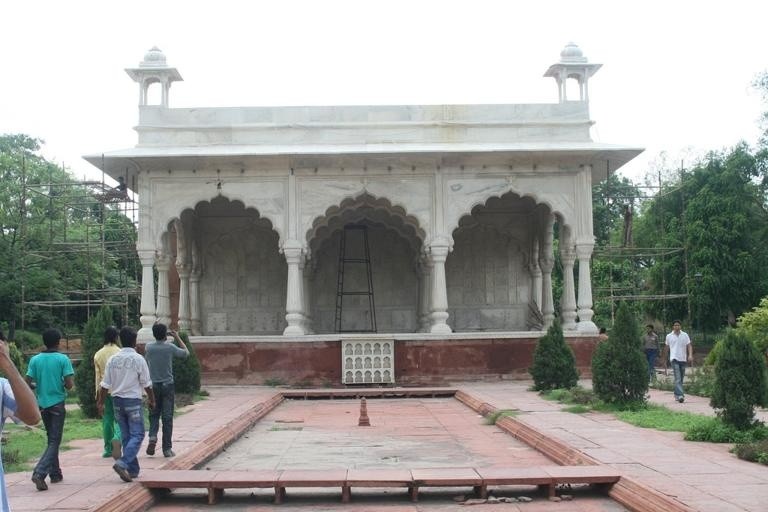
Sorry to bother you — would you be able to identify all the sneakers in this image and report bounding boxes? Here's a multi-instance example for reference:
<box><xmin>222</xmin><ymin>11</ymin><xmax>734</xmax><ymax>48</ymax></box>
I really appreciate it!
<box><xmin>113</xmin><ymin>464</ymin><xmax>132</xmax><ymax>482</ymax></box>
<box><xmin>111</xmin><ymin>440</ymin><xmax>121</xmax><ymax>460</ymax></box>
<box><xmin>146</xmin><ymin>439</ymin><xmax>156</xmax><ymax>455</ymax></box>
<box><xmin>50</xmin><ymin>474</ymin><xmax>62</xmax><ymax>483</ymax></box>
<box><xmin>163</xmin><ymin>449</ymin><xmax>176</xmax><ymax>457</ymax></box>
<box><xmin>32</xmin><ymin>472</ymin><xmax>47</xmax><ymax>490</ymax></box>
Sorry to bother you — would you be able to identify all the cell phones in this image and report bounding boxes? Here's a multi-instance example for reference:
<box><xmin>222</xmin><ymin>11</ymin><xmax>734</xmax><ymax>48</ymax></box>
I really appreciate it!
<box><xmin>166</xmin><ymin>332</ymin><xmax>172</xmax><ymax>336</ymax></box>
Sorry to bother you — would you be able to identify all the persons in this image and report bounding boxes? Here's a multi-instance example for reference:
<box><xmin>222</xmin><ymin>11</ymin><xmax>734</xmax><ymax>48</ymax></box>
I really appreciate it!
<box><xmin>662</xmin><ymin>320</ymin><xmax>693</xmax><ymax>403</ymax></box>
<box><xmin>98</xmin><ymin>327</ymin><xmax>155</xmax><ymax>482</ymax></box>
<box><xmin>145</xmin><ymin>323</ymin><xmax>189</xmax><ymax>458</ymax></box>
<box><xmin>94</xmin><ymin>327</ymin><xmax>121</xmax><ymax>460</ymax></box>
<box><xmin>622</xmin><ymin>204</ymin><xmax>631</xmax><ymax>247</ymax></box>
<box><xmin>24</xmin><ymin>328</ymin><xmax>74</xmax><ymax>490</ymax></box>
<box><xmin>641</xmin><ymin>325</ymin><xmax>661</xmax><ymax>383</ymax></box>
<box><xmin>105</xmin><ymin>176</ymin><xmax>127</xmax><ymax>200</ymax></box>
<box><xmin>599</xmin><ymin>327</ymin><xmax>608</xmax><ymax>342</ymax></box>
<box><xmin>0</xmin><ymin>332</ymin><xmax>42</xmax><ymax>512</ymax></box>
